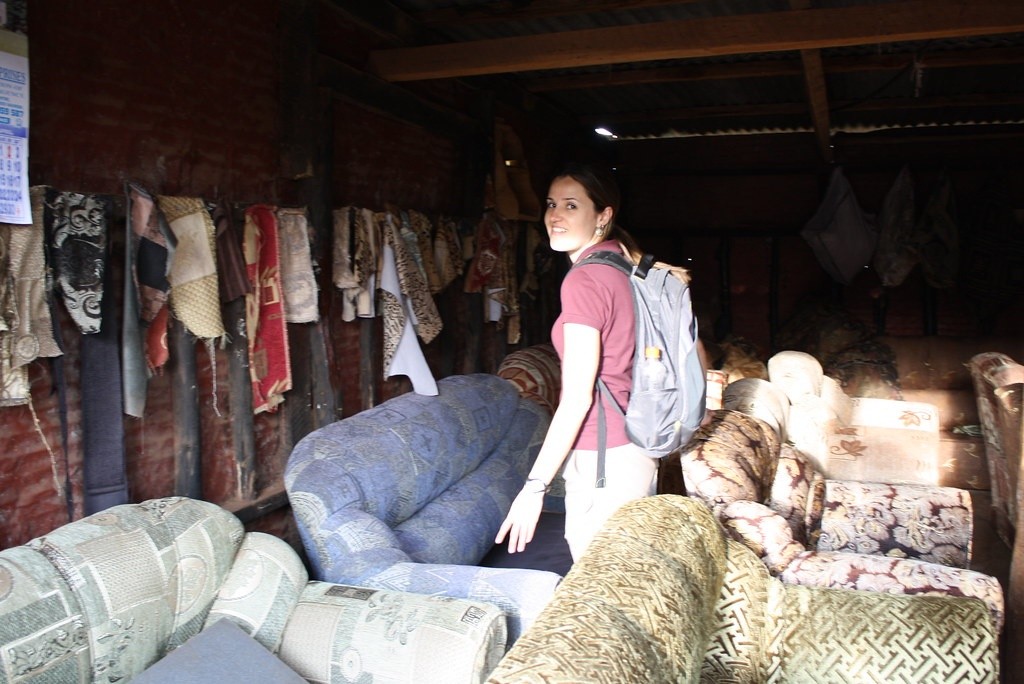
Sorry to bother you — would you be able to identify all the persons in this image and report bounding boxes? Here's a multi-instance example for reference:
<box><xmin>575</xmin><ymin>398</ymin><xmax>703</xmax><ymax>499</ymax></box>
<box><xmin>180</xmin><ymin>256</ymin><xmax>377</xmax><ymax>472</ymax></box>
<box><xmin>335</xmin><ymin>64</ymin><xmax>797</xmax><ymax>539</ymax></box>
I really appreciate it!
<box><xmin>493</xmin><ymin>166</ymin><xmax>660</xmax><ymax>569</ymax></box>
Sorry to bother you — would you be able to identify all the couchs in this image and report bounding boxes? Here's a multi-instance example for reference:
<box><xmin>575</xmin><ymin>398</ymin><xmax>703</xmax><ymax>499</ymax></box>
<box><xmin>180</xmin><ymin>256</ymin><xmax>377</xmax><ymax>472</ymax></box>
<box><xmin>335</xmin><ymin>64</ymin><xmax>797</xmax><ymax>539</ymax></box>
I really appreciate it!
<box><xmin>0</xmin><ymin>325</ymin><xmax>1024</xmax><ymax>684</ymax></box>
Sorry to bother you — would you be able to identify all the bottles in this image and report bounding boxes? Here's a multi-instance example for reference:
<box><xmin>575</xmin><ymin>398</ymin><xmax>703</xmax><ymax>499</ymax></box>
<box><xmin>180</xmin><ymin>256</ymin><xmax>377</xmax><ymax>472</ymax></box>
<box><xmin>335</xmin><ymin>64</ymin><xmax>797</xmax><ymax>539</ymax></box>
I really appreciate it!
<box><xmin>639</xmin><ymin>346</ymin><xmax>666</xmax><ymax>392</ymax></box>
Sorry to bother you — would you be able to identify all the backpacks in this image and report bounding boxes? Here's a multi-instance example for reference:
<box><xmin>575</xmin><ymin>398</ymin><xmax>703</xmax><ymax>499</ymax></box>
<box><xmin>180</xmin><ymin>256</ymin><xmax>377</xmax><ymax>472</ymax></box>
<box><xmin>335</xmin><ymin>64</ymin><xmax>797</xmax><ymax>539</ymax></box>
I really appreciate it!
<box><xmin>573</xmin><ymin>251</ymin><xmax>707</xmax><ymax>458</ymax></box>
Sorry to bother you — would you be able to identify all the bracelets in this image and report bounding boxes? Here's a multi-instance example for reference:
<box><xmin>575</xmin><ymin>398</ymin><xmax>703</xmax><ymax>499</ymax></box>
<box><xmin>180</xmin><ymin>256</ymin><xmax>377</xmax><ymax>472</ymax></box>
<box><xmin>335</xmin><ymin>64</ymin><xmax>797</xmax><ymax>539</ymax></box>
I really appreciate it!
<box><xmin>518</xmin><ymin>477</ymin><xmax>554</xmax><ymax>495</ymax></box>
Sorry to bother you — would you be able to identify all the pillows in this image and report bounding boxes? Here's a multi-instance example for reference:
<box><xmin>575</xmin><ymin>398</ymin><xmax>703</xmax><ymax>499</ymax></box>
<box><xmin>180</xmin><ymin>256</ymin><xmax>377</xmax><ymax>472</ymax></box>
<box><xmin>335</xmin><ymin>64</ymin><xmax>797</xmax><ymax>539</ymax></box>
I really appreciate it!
<box><xmin>127</xmin><ymin>617</ymin><xmax>311</xmax><ymax>684</ymax></box>
